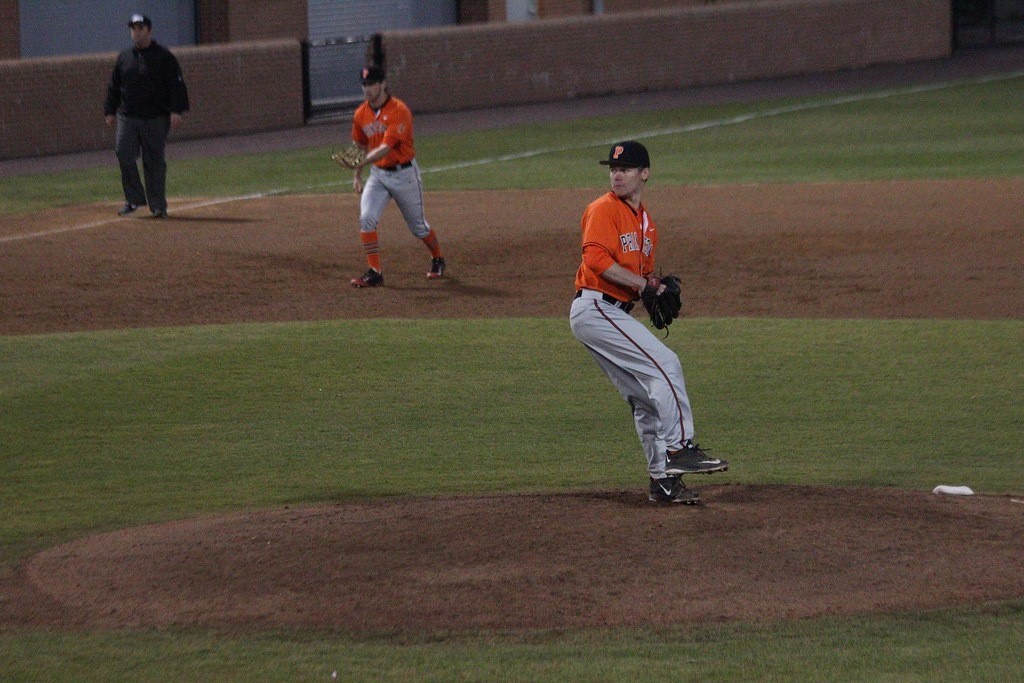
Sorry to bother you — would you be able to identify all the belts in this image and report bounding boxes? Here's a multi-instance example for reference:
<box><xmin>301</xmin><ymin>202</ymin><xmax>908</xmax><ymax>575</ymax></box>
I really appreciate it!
<box><xmin>377</xmin><ymin>160</ymin><xmax>413</xmax><ymax>172</ymax></box>
<box><xmin>572</xmin><ymin>288</ymin><xmax>636</xmax><ymax>314</ymax></box>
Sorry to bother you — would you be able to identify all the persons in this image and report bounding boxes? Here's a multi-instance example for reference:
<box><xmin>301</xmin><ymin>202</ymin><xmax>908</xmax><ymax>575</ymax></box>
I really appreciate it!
<box><xmin>102</xmin><ymin>12</ymin><xmax>191</xmax><ymax>219</ymax></box>
<box><xmin>570</xmin><ymin>139</ymin><xmax>731</xmax><ymax>507</ymax></box>
<box><xmin>331</xmin><ymin>65</ymin><xmax>447</xmax><ymax>290</ymax></box>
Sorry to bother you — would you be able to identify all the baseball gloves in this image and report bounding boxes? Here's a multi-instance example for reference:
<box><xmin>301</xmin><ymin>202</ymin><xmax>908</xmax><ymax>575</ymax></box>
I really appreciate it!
<box><xmin>641</xmin><ymin>266</ymin><xmax>683</xmax><ymax>341</ymax></box>
<box><xmin>330</xmin><ymin>142</ymin><xmax>364</xmax><ymax>169</ymax></box>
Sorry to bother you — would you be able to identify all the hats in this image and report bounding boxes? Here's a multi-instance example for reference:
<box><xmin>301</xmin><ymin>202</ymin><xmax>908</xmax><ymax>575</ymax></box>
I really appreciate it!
<box><xmin>360</xmin><ymin>65</ymin><xmax>385</xmax><ymax>86</ymax></box>
<box><xmin>127</xmin><ymin>13</ymin><xmax>153</xmax><ymax>27</ymax></box>
<box><xmin>599</xmin><ymin>140</ymin><xmax>653</xmax><ymax>169</ymax></box>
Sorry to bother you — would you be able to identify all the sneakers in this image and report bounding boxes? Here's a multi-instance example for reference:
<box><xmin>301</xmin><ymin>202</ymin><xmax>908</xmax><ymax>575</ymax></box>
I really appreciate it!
<box><xmin>350</xmin><ymin>267</ymin><xmax>383</xmax><ymax>289</ymax></box>
<box><xmin>647</xmin><ymin>474</ymin><xmax>703</xmax><ymax>505</ymax></box>
<box><xmin>151</xmin><ymin>205</ymin><xmax>169</xmax><ymax>219</ymax></box>
<box><xmin>426</xmin><ymin>257</ymin><xmax>447</xmax><ymax>279</ymax></box>
<box><xmin>117</xmin><ymin>201</ymin><xmax>147</xmax><ymax>217</ymax></box>
<box><xmin>662</xmin><ymin>437</ymin><xmax>733</xmax><ymax>478</ymax></box>
